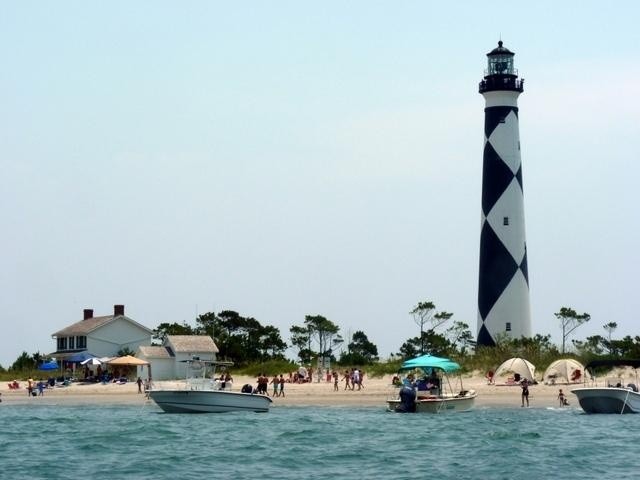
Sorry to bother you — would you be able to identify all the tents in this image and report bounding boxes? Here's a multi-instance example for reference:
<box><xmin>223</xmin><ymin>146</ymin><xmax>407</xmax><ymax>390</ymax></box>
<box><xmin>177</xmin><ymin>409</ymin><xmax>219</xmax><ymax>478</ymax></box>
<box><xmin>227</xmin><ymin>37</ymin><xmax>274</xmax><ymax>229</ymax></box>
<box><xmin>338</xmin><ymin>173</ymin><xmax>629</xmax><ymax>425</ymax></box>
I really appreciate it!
<box><xmin>543</xmin><ymin>358</ymin><xmax>592</xmax><ymax>387</ymax></box>
<box><xmin>493</xmin><ymin>358</ymin><xmax>536</xmax><ymax>384</ymax></box>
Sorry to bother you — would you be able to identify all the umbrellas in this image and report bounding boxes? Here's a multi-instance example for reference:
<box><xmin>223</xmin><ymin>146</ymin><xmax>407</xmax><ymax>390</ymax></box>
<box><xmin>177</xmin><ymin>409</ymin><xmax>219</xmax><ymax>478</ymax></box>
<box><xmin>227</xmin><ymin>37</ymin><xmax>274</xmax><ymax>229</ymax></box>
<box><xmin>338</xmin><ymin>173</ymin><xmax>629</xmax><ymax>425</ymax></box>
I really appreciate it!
<box><xmin>39</xmin><ymin>362</ymin><xmax>59</xmax><ymax>370</ymax></box>
<box><xmin>67</xmin><ymin>350</ymin><xmax>151</xmax><ymax>381</ymax></box>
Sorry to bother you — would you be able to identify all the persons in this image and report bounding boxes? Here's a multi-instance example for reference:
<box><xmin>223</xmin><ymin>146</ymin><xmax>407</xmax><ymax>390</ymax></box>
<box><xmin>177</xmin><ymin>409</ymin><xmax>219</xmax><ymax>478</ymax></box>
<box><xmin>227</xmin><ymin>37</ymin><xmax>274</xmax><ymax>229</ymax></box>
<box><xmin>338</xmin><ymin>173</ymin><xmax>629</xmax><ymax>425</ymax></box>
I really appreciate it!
<box><xmin>220</xmin><ymin>362</ymin><xmax>365</xmax><ymax>398</ymax></box>
<box><xmin>570</xmin><ymin>366</ymin><xmax>582</xmax><ymax>382</ymax></box>
<box><xmin>391</xmin><ymin>368</ymin><xmax>442</xmax><ymax>413</ymax></box>
<box><xmin>557</xmin><ymin>389</ymin><xmax>565</xmax><ymax>407</ymax></box>
<box><xmin>11</xmin><ymin>365</ymin><xmax>156</xmax><ymax>400</ymax></box>
<box><xmin>521</xmin><ymin>377</ymin><xmax>529</xmax><ymax>407</ymax></box>
<box><xmin>486</xmin><ymin>369</ymin><xmax>493</xmax><ymax>384</ymax></box>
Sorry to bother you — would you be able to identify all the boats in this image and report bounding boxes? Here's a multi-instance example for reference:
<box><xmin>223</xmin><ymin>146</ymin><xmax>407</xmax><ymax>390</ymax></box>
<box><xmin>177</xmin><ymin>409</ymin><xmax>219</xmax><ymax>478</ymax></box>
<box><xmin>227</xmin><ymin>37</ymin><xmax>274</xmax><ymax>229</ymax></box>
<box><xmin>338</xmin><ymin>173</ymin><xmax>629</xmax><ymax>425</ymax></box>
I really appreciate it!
<box><xmin>387</xmin><ymin>361</ymin><xmax>477</xmax><ymax>414</ymax></box>
<box><xmin>145</xmin><ymin>357</ymin><xmax>272</xmax><ymax>418</ymax></box>
<box><xmin>570</xmin><ymin>385</ymin><xmax>640</xmax><ymax>416</ymax></box>
